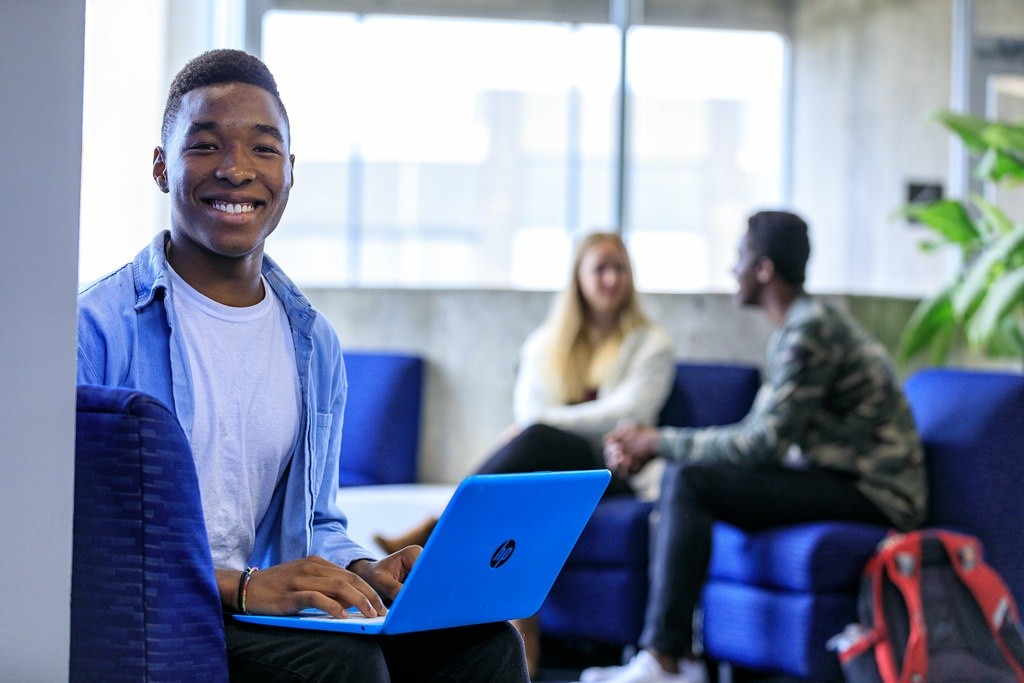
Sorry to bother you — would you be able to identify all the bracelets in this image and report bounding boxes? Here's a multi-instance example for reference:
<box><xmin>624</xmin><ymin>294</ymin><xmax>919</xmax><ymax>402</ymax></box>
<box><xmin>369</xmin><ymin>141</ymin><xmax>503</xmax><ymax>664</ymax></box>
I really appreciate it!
<box><xmin>237</xmin><ymin>566</ymin><xmax>259</xmax><ymax>614</ymax></box>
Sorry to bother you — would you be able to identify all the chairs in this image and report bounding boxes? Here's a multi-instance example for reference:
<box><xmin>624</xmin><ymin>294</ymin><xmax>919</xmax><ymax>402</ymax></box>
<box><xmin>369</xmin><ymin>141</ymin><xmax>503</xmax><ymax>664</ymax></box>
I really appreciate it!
<box><xmin>69</xmin><ymin>386</ymin><xmax>228</xmax><ymax>683</ymax></box>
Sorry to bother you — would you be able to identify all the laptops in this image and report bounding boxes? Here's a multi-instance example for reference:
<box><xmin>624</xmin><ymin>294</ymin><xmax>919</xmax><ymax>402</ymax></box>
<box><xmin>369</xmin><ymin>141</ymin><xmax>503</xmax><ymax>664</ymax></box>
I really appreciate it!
<box><xmin>232</xmin><ymin>469</ymin><xmax>613</xmax><ymax>635</ymax></box>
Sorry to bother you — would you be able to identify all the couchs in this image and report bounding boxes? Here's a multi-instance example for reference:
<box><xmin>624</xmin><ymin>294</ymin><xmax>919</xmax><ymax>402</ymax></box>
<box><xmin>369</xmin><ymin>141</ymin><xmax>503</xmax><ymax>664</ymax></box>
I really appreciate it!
<box><xmin>693</xmin><ymin>366</ymin><xmax>1024</xmax><ymax>683</ymax></box>
<box><xmin>539</xmin><ymin>364</ymin><xmax>761</xmax><ymax>663</ymax></box>
<box><xmin>336</xmin><ymin>352</ymin><xmax>425</xmax><ymax>485</ymax></box>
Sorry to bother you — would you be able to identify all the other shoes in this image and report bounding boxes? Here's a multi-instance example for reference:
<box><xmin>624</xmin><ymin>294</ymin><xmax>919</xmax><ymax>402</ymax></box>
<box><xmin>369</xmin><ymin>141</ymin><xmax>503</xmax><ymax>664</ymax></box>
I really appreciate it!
<box><xmin>580</xmin><ymin>652</ymin><xmax>706</xmax><ymax>683</ymax></box>
<box><xmin>373</xmin><ymin>517</ymin><xmax>440</xmax><ymax>553</ymax></box>
<box><xmin>509</xmin><ymin>611</ymin><xmax>539</xmax><ymax>678</ymax></box>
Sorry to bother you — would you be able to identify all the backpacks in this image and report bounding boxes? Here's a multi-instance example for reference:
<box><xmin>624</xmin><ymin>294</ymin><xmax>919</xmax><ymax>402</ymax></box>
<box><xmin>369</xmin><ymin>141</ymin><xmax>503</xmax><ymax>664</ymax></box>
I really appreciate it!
<box><xmin>825</xmin><ymin>525</ymin><xmax>1024</xmax><ymax>683</ymax></box>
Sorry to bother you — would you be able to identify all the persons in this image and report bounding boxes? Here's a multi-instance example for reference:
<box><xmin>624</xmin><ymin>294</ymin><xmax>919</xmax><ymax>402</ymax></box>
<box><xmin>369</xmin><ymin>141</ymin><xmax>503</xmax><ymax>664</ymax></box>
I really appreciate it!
<box><xmin>371</xmin><ymin>232</ymin><xmax>676</xmax><ymax>555</ymax></box>
<box><xmin>579</xmin><ymin>211</ymin><xmax>930</xmax><ymax>683</ymax></box>
<box><xmin>78</xmin><ymin>49</ymin><xmax>530</xmax><ymax>683</ymax></box>
